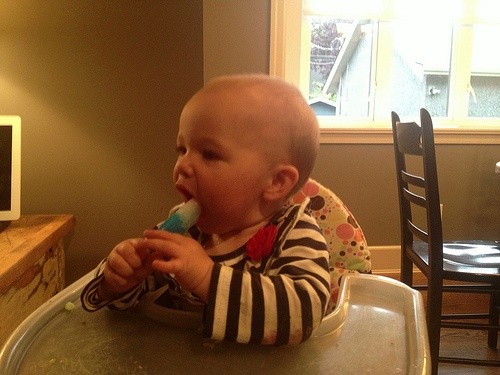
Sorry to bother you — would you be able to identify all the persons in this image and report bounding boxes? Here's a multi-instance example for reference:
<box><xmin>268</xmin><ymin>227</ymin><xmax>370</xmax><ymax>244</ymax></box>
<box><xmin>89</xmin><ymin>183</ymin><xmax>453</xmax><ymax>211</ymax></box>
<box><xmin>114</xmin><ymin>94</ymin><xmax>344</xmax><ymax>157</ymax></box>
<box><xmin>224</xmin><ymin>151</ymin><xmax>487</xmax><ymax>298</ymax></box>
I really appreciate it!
<box><xmin>80</xmin><ymin>73</ymin><xmax>372</xmax><ymax>348</ymax></box>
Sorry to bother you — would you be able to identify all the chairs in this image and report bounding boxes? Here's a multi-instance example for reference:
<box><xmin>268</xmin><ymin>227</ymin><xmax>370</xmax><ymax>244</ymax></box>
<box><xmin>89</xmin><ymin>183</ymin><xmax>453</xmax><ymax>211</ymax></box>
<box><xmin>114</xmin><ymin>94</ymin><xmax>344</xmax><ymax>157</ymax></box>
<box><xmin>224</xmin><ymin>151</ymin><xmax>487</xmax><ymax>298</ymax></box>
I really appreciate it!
<box><xmin>168</xmin><ymin>177</ymin><xmax>374</xmax><ymax>319</ymax></box>
<box><xmin>391</xmin><ymin>107</ymin><xmax>499</xmax><ymax>374</ymax></box>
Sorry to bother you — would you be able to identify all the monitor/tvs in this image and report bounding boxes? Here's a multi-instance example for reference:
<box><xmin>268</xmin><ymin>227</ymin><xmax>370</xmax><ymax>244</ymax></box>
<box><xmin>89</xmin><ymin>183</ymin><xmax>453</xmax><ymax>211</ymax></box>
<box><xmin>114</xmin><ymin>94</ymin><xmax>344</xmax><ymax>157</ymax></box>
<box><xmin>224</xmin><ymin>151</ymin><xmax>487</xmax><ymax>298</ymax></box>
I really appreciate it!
<box><xmin>0</xmin><ymin>114</ymin><xmax>21</xmax><ymax>221</ymax></box>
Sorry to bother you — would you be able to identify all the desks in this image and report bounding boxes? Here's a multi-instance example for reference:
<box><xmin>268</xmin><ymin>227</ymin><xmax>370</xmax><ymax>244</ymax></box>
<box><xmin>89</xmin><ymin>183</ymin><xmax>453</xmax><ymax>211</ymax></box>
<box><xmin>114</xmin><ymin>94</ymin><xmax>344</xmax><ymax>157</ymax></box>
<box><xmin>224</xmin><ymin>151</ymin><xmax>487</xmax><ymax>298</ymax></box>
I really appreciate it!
<box><xmin>1</xmin><ymin>211</ymin><xmax>76</xmax><ymax>353</ymax></box>
